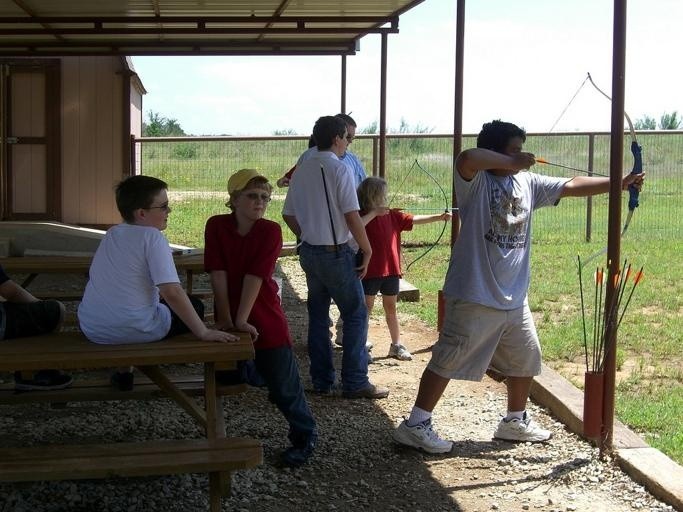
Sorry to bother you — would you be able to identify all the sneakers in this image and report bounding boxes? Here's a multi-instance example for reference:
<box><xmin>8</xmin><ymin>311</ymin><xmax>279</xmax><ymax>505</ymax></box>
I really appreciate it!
<box><xmin>387</xmin><ymin>342</ymin><xmax>412</xmax><ymax>361</ymax></box>
<box><xmin>13</xmin><ymin>368</ymin><xmax>73</xmax><ymax>392</ymax></box>
<box><xmin>340</xmin><ymin>382</ymin><xmax>389</xmax><ymax>399</ymax></box>
<box><xmin>108</xmin><ymin>370</ymin><xmax>134</xmax><ymax>391</ymax></box>
<box><xmin>333</xmin><ymin>333</ymin><xmax>373</xmax><ymax>351</ymax></box>
<box><xmin>492</xmin><ymin>410</ymin><xmax>553</xmax><ymax>444</ymax></box>
<box><xmin>279</xmin><ymin>446</ymin><xmax>312</xmax><ymax>466</ymax></box>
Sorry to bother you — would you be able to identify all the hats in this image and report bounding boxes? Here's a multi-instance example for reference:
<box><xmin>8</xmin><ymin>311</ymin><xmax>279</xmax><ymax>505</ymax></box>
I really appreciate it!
<box><xmin>225</xmin><ymin>168</ymin><xmax>270</xmax><ymax>195</ymax></box>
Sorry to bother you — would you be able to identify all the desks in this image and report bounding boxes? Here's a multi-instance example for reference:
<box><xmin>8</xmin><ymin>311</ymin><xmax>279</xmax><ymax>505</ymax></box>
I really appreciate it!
<box><xmin>0</xmin><ymin>253</ymin><xmax>208</xmax><ymax>296</ymax></box>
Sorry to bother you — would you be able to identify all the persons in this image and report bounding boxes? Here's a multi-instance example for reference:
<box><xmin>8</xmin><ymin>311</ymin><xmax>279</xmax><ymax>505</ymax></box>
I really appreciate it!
<box><xmin>77</xmin><ymin>175</ymin><xmax>241</xmax><ymax>392</ymax></box>
<box><xmin>0</xmin><ymin>265</ymin><xmax>75</xmax><ymax>393</ymax></box>
<box><xmin>203</xmin><ymin>168</ymin><xmax>320</xmax><ymax>466</ymax></box>
<box><xmin>276</xmin><ymin>112</ymin><xmax>390</xmax><ymax>400</ymax></box>
<box><xmin>355</xmin><ymin>177</ymin><xmax>453</xmax><ymax>361</ymax></box>
<box><xmin>390</xmin><ymin>119</ymin><xmax>646</xmax><ymax>455</ymax></box>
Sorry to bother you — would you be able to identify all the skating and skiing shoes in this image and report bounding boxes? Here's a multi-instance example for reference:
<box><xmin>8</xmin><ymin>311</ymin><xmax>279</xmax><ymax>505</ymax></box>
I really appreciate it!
<box><xmin>391</xmin><ymin>416</ymin><xmax>453</xmax><ymax>455</ymax></box>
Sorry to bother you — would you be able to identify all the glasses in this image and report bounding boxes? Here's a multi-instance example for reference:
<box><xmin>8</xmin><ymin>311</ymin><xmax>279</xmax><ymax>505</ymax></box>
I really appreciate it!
<box><xmin>141</xmin><ymin>203</ymin><xmax>168</xmax><ymax>212</ymax></box>
<box><xmin>240</xmin><ymin>192</ymin><xmax>271</xmax><ymax>201</ymax></box>
